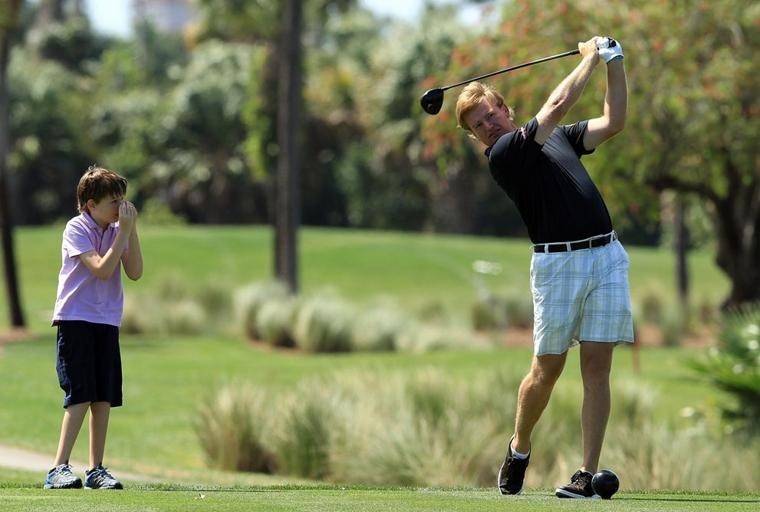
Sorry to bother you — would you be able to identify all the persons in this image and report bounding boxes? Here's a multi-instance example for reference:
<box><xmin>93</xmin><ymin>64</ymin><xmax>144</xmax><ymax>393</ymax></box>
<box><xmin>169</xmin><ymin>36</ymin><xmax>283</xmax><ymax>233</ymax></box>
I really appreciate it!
<box><xmin>455</xmin><ymin>36</ymin><xmax>635</xmax><ymax>498</ymax></box>
<box><xmin>44</xmin><ymin>165</ymin><xmax>143</xmax><ymax>490</ymax></box>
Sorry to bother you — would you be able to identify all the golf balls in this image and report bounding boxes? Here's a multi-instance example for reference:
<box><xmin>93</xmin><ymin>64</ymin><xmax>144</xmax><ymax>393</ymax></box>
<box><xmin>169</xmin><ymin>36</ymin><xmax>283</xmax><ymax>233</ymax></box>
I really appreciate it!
<box><xmin>592</xmin><ymin>470</ymin><xmax>619</xmax><ymax>497</ymax></box>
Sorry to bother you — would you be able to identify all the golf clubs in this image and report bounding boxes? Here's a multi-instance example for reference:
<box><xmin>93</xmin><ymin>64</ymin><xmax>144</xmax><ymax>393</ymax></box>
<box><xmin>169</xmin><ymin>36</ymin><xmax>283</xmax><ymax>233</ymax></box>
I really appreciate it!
<box><xmin>420</xmin><ymin>38</ymin><xmax>616</xmax><ymax>115</ymax></box>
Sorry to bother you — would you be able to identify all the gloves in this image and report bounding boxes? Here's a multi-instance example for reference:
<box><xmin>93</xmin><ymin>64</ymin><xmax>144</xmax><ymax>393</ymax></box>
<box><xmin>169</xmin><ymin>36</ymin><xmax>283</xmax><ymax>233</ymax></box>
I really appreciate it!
<box><xmin>594</xmin><ymin>36</ymin><xmax>624</xmax><ymax>64</ymax></box>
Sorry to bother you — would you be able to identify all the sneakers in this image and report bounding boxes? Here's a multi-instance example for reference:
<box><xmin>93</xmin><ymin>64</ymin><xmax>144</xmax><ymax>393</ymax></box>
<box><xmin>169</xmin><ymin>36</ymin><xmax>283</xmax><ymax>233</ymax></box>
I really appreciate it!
<box><xmin>43</xmin><ymin>463</ymin><xmax>82</xmax><ymax>489</ymax></box>
<box><xmin>556</xmin><ymin>468</ymin><xmax>600</xmax><ymax>499</ymax></box>
<box><xmin>85</xmin><ymin>464</ymin><xmax>122</xmax><ymax>490</ymax></box>
<box><xmin>498</xmin><ymin>436</ymin><xmax>531</xmax><ymax>495</ymax></box>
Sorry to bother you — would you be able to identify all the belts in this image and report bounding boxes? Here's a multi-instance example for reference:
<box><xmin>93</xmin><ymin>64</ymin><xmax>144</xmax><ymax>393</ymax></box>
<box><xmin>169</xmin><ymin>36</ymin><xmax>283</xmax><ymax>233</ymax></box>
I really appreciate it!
<box><xmin>534</xmin><ymin>232</ymin><xmax>617</xmax><ymax>253</ymax></box>
